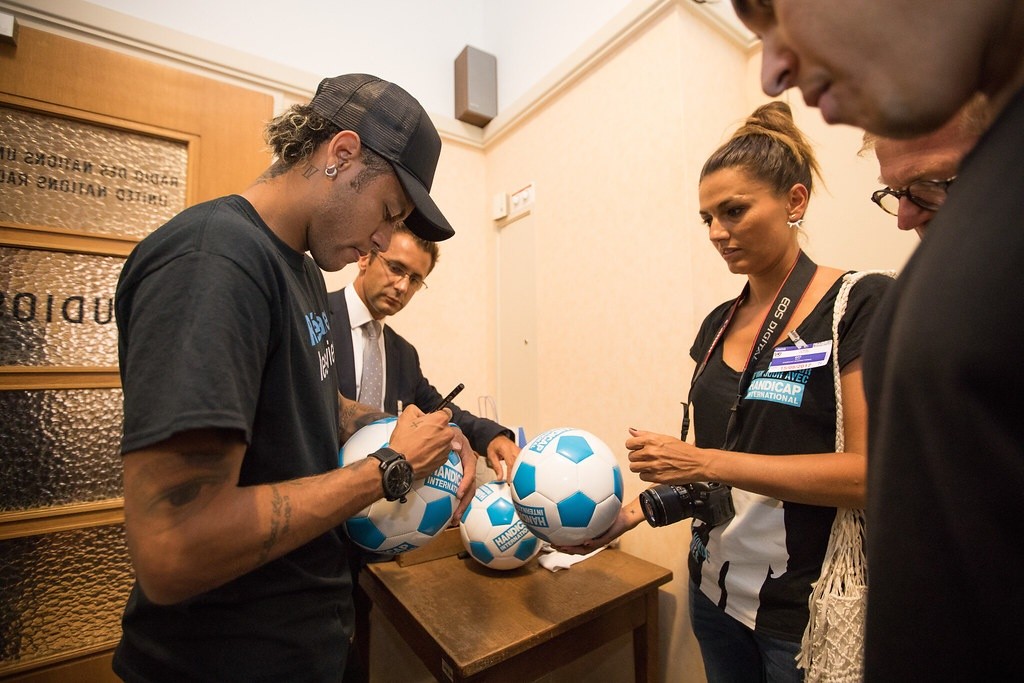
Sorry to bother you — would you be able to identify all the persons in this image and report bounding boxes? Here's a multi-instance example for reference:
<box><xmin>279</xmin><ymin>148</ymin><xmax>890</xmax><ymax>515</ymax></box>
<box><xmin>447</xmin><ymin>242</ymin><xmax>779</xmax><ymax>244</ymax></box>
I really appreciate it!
<box><xmin>112</xmin><ymin>74</ymin><xmax>456</xmax><ymax>683</ymax></box>
<box><xmin>550</xmin><ymin>101</ymin><xmax>899</xmax><ymax>683</ymax></box>
<box><xmin>855</xmin><ymin>90</ymin><xmax>992</xmax><ymax>243</ymax></box>
<box><xmin>730</xmin><ymin>0</ymin><xmax>1024</xmax><ymax>683</ymax></box>
<box><xmin>327</xmin><ymin>222</ymin><xmax>522</xmax><ymax>487</ymax></box>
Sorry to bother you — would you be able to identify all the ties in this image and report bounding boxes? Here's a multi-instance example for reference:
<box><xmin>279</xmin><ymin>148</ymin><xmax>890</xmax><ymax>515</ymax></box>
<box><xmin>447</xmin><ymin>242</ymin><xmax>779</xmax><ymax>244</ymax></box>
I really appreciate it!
<box><xmin>356</xmin><ymin>319</ymin><xmax>384</xmax><ymax>412</ymax></box>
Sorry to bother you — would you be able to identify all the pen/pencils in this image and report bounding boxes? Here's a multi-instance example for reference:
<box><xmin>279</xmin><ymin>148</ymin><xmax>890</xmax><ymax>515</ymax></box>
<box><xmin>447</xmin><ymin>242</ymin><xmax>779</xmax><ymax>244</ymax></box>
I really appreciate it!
<box><xmin>426</xmin><ymin>383</ymin><xmax>465</xmax><ymax>413</ymax></box>
<box><xmin>457</xmin><ymin>550</ymin><xmax>471</xmax><ymax>559</ymax></box>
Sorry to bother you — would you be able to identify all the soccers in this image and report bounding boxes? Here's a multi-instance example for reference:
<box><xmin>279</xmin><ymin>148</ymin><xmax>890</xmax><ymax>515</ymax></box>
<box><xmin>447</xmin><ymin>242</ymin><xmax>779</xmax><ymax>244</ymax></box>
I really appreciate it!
<box><xmin>511</xmin><ymin>428</ymin><xmax>624</xmax><ymax>547</ymax></box>
<box><xmin>340</xmin><ymin>416</ymin><xmax>463</xmax><ymax>555</ymax></box>
<box><xmin>461</xmin><ymin>479</ymin><xmax>545</xmax><ymax>570</ymax></box>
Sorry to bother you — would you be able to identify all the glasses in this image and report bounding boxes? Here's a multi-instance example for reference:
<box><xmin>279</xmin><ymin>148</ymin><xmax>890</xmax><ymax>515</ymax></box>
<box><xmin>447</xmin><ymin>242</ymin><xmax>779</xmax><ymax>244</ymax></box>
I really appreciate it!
<box><xmin>368</xmin><ymin>250</ymin><xmax>429</xmax><ymax>292</ymax></box>
<box><xmin>872</xmin><ymin>172</ymin><xmax>960</xmax><ymax>218</ymax></box>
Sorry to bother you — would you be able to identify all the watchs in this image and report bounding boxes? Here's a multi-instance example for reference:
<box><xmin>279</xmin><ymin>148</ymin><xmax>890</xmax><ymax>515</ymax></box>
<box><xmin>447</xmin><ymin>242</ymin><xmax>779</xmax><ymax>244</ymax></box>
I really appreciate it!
<box><xmin>367</xmin><ymin>448</ymin><xmax>414</xmax><ymax>502</ymax></box>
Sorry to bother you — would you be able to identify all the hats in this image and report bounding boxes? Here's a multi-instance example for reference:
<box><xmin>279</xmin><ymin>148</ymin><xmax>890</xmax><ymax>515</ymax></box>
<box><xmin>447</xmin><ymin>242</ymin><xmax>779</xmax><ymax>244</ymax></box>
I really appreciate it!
<box><xmin>306</xmin><ymin>74</ymin><xmax>455</xmax><ymax>242</ymax></box>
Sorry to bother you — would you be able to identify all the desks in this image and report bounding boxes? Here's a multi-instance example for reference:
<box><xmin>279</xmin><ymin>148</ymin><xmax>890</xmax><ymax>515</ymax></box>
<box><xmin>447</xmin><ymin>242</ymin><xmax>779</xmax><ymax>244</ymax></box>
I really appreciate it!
<box><xmin>350</xmin><ymin>548</ymin><xmax>673</xmax><ymax>683</ymax></box>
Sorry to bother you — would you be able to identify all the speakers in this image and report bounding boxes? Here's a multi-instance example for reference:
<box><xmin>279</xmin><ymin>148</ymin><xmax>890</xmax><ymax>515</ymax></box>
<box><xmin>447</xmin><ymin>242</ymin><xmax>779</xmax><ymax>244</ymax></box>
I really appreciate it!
<box><xmin>453</xmin><ymin>44</ymin><xmax>496</xmax><ymax>129</ymax></box>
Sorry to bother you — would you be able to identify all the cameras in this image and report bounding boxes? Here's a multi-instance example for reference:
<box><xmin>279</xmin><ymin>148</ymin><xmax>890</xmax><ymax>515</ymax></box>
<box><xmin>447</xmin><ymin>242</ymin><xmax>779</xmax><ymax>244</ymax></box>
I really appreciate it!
<box><xmin>640</xmin><ymin>473</ymin><xmax>735</xmax><ymax>528</ymax></box>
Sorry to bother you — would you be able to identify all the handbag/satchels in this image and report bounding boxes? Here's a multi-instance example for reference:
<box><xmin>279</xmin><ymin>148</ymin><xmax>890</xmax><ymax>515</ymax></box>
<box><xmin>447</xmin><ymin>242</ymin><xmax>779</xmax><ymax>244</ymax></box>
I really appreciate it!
<box><xmin>797</xmin><ymin>504</ymin><xmax>868</xmax><ymax>683</ymax></box>
